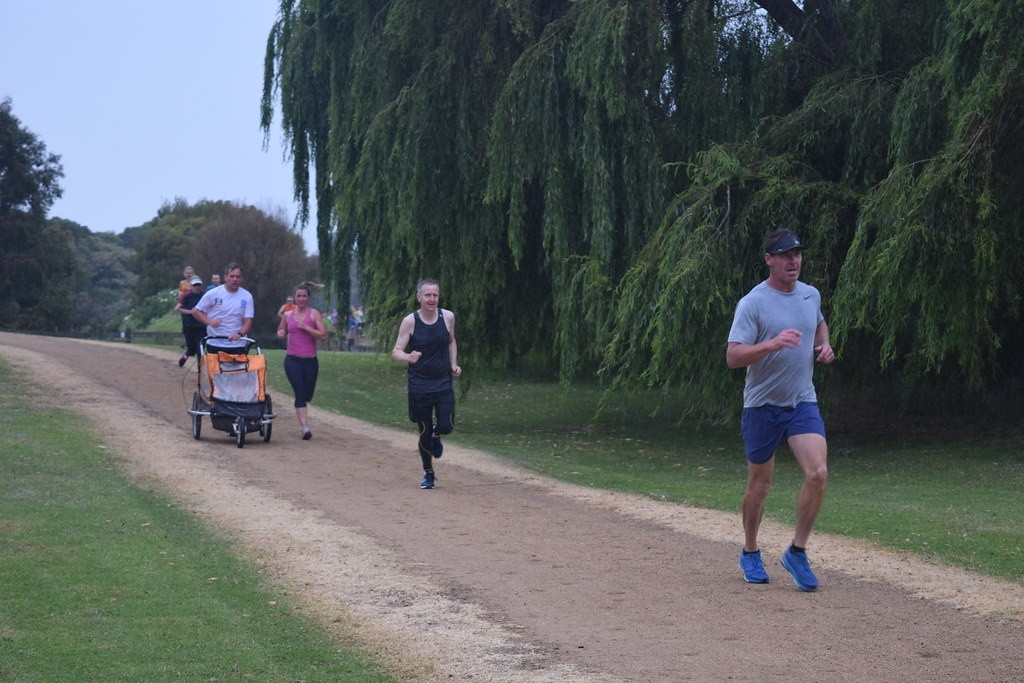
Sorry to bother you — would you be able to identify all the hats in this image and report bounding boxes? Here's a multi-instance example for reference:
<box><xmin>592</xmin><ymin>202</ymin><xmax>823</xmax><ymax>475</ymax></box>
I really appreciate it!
<box><xmin>767</xmin><ymin>235</ymin><xmax>808</xmax><ymax>255</ymax></box>
<box><xmin>191</xmin><ymin>279</ymin><xmax>203</xmax><ymax>285</ymax></box>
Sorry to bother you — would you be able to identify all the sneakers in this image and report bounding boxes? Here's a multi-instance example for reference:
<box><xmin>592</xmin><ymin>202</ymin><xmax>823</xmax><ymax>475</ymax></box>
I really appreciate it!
<box><xmin>431</xmin><ymin>424</ymin><xmax>443</xmax><ymax>457</ymax></box>
<box><xmin>780</xmin><ymin>541</ymin><xmax>818</xmax><ymax>591</ymax></box>
<box><xmin>421</xmin><ymin>470</ymin><xmax>436</xmax><ymax>489</ymax></box>
<box><xmin>739</xmin><ymin>546</ymin><xmax>770</xmax><ymax>583</ymax></box>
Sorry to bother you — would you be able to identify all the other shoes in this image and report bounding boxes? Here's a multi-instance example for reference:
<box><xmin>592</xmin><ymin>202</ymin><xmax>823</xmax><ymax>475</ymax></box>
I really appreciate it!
<box><xmin>178</xmin><ymin>353</ymin><xmax>188</xmax><ymax>367</ymax></box>
<box><xmin>300</xmin><ymin>427</ymin><xmax>312</xmax><ymax>439</ymax></box>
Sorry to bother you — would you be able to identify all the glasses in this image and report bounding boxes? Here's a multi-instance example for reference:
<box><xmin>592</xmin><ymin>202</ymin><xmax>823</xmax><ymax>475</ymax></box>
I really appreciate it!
<box><xmin>193</xmin><ymin>284</ymin><xmax>202</xmax><ymax>287</ymax></box>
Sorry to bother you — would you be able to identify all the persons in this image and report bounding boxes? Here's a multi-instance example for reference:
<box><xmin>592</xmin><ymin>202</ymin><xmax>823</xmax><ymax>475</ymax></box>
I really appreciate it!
<box><xmin>175</xmin><ymin>276</ymin><xmax>207</xmax><ymax>374</ymax></box>
<box><xmin>320</xmin><ymin>305</ymin><xmax>366</xmax><ymax>351</ymax></box>
<box><xmin>207</xmin><ymin>273</ymin><xmax>221</xmax><ymax>291</ymax></box>
<box><xmin>277</xmin><ymin>281</ymin><xmax>327</xmax><ymax>439</ymax></box>
<box><xmin>191</xmin><ymin>263</ymin><xmax>254</xmax><ymax>354</ymax></box>
<box><xmin>727</xmin><ymin>228</ymin><xmax>835</xmax><ymax>591</ymax></box>
<box><xmin>178</xmin><ymin>265</ymin><xmax>195</xmax><ymax>298</ymax></box>
<box><xmin>219</xmin><ymin>272</ymin><xmax>227</xmax><ymax>287</ymax></box>
<box><xmin>277</xmin><ymin>295</ymin><xmax>297</xmax><ymax>317</ymax></box>
<box><xmin>392</xmin><ymin>280</ymin><xmax>462</xmax><ymax>490</ymax></box>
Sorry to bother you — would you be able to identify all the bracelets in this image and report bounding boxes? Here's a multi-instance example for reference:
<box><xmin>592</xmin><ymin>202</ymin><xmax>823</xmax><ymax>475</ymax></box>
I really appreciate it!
<box><xmin>237</xmin><ymin>332</ymin><xmax>244</xmax><ymax>337</ymax></box>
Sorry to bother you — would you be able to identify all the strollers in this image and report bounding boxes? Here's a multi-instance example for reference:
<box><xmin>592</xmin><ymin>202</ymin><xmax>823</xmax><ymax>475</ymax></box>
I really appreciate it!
<box><xmin>191</xmin><ymin>335</ymin><xmax>274</xmax><ymax>448</ymax></box>
